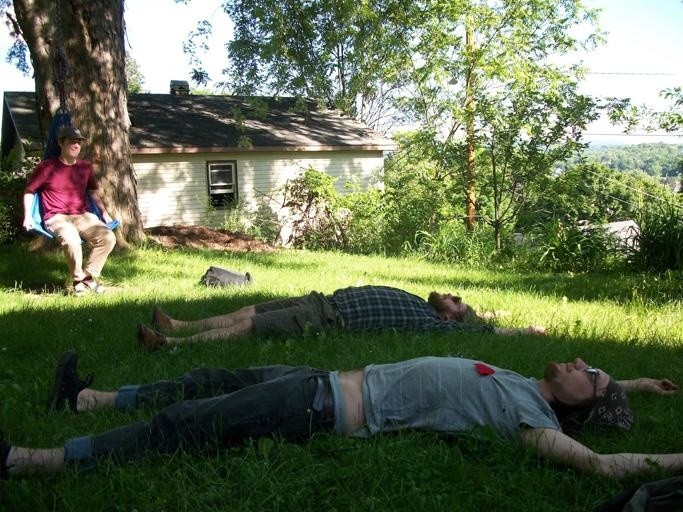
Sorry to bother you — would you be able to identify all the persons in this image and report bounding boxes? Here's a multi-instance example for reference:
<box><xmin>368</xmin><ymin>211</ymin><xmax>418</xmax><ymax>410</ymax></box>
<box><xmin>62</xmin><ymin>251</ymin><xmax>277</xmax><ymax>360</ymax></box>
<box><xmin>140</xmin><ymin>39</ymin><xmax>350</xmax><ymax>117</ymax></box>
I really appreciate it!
<box><xmin>135</xmin><ymin>284</ymin><xmax>548</xmax><ymax>354</ymax></box>
<box><xmin>0</xmin><ymin>349</ymin><xmax>681</xmax><ymax>486</ymax></box>
<box><xmin>21</xmin><ymin>124</ymin><xmax>114</xmax><ymax>295</ymax></box>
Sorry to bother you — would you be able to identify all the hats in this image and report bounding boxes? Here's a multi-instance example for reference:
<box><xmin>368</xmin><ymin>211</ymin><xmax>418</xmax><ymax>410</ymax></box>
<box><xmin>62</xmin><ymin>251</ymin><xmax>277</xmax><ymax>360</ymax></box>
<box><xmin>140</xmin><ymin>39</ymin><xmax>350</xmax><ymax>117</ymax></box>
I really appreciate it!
<box><xmin>57</xmin><ymin>126</ymin><xmax>85</xmax><ymax>141</ymax></box>
<box><xmin>586</xmin><ymin>377</ymin><xmax>634</xmax><ymax>432</ymax></box>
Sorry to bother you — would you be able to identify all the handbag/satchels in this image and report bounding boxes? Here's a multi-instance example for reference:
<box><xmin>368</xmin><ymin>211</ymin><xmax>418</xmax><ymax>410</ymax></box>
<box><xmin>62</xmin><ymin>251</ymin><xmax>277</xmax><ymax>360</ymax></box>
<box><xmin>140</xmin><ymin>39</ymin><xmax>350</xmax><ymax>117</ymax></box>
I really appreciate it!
<box><xmin>197</xmin><ymin>267</ymin><xmax>252</xmax><ymax>287</ymax></box>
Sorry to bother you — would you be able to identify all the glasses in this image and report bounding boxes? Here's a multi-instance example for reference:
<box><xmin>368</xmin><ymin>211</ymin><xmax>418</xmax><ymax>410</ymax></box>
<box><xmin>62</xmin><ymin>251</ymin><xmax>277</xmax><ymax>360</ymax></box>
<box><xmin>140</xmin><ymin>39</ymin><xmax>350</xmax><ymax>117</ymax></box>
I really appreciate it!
<box><xmin>451</xmin><ymin>295</ymin><xmax>461</xmax><ymax>319</ymax></box>
<box><xmin>584</xmin><ymin>364</ymin><xmax>600</xmax><ymax>400</ymax></box>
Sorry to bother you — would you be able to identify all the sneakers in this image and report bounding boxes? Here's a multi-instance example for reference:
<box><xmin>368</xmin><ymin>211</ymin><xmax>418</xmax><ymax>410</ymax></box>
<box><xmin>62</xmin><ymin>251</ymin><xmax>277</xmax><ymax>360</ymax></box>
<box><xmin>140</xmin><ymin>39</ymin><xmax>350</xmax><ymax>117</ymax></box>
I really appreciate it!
<box><xmin>135</xmin><ymin>323</ymin><xmax>166</xmax><ymax>357</ymax></box>
<box><xmin>43</xmin><ymin>350</ymin><xmax>91</xmax><ymax>419</ymax></box>
<box><xmin>82</xmin><ymin>276</ymin><xmax>106</xmax><ymax>295</ymax></box>
<box><xmin>72</xmin><ymin>280</ymin><xmax>86</xmax><ymax>298</ymax></box>
<box><xmin>0</xmin><ymin>430</ymin><xmax>12</xmax><ymax>481</ymax></box>
<box><xmin>152</xmin><ymin>304</ymin><xmax>173</xmax><ymax>332</ymax></box>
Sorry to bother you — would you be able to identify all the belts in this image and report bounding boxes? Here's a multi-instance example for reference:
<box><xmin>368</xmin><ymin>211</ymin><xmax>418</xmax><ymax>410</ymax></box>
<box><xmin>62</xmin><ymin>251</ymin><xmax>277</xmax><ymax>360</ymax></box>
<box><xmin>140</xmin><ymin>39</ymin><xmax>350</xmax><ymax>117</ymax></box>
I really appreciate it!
<box><xmin>313</xmin><ymin>369</ymin><xmax>334</xmax><ymax>433</ymax></box>
